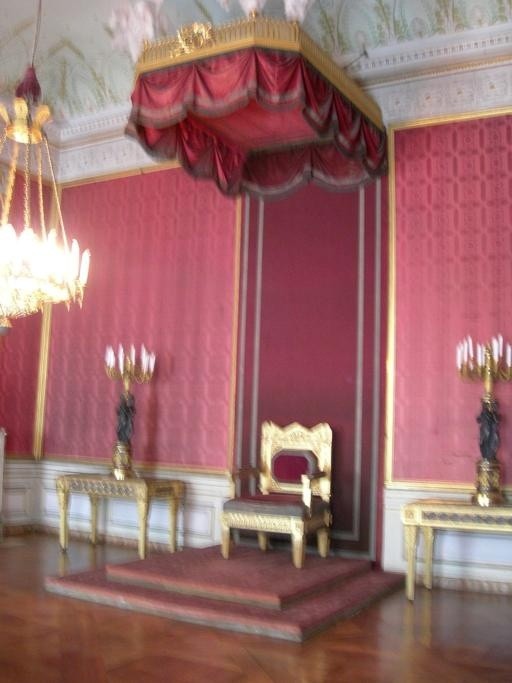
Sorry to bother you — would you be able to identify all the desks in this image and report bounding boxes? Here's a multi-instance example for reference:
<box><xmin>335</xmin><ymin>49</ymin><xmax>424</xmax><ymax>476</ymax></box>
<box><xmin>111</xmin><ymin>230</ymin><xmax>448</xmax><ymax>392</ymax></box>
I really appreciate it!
<box><xmin>56</xmin><ymin>472</ymin><xmax>185</xmax><ymax>560</ymax></box>
<box><xmin>402</xmin><ymin>497</ymin><xmax>512</xmax><ymax>600</ymax></box>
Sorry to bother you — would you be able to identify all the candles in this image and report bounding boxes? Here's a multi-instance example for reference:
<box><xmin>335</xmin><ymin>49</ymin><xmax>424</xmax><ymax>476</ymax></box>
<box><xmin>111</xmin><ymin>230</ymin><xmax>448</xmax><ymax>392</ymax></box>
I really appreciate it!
<box><xmin>454</xmin><ymin>333</ymin><xmax>511</xmax><ymax>371</ymax></box>
<box><xmin>103</xmin><ymin>342</ymin><xmax>157</xmax><ymax>374</ymax></box>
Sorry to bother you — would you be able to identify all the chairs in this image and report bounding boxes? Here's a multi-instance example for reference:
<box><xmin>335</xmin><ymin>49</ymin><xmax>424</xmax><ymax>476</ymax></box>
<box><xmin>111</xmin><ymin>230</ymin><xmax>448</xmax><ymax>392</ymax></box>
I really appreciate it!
<box><xmin>221</xmin><ymin>420</ymin><xmax>333</xmax><ymax>570</ymax></box>
